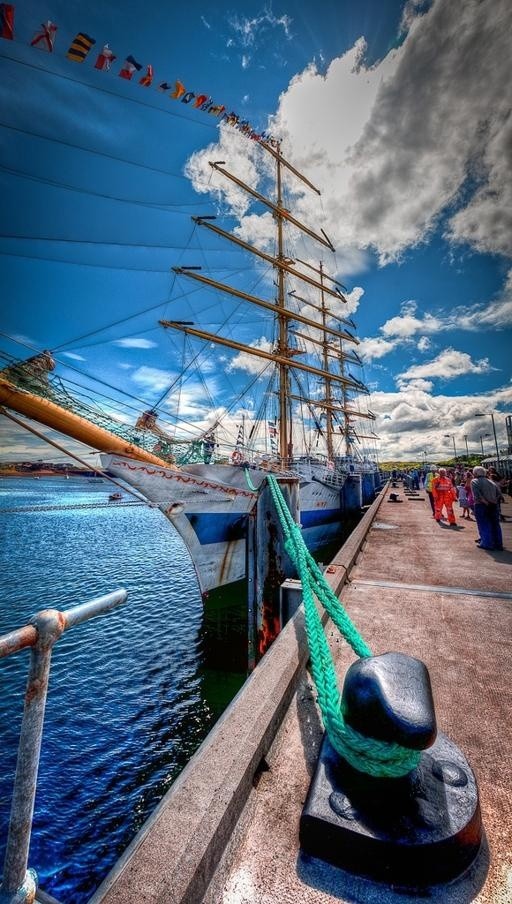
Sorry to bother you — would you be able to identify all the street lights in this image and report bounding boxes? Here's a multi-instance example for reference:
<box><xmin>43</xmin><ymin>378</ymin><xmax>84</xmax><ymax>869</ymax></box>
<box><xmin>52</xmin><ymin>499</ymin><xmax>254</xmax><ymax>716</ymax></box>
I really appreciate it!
<box><xmin>443</xmin><ymin>414</ymin><xmax>501</xmax><ymax>471</ymax></box>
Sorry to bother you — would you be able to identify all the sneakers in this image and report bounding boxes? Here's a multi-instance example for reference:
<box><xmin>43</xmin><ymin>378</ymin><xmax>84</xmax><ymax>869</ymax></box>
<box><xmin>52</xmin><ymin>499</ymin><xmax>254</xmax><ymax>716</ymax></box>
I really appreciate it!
<box><xmin>459</xmin><ymin>515</ymin><xmax>470</xmax><ymax>519</ymax></box>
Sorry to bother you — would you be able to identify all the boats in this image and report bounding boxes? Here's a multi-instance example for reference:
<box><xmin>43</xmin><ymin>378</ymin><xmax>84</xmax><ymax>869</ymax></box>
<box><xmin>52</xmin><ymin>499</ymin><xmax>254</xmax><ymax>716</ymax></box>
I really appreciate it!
<box><xmin>109</xmin><ymin>492</ymin><xmax>121</xmax><ymax>499</ymax></box>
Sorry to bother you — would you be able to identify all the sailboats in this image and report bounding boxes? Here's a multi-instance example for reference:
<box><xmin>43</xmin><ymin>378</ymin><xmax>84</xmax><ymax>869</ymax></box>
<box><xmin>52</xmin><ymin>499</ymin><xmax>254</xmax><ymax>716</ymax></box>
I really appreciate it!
<box><xmin>0</xmin><ymin>138</ymin><xmax>379</xmax><ymax>610</ymax></box>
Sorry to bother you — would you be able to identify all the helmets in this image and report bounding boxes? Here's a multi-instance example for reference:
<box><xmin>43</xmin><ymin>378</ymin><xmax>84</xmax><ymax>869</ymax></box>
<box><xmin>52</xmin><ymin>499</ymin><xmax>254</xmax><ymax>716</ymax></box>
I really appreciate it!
<box><xmin>430</xmin><ymin>465</ymin><xmax>438</xmax><ymax>470</ymax></box>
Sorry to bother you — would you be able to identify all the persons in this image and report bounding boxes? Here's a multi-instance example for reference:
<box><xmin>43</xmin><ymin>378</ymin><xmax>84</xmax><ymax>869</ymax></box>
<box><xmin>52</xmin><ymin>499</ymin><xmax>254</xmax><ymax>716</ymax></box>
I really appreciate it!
<box><xmin>390</xmin><ymin>467</ymin><xmax>422</xmax><ymax>491</ymax></box>
<box><xmin>422</xmin><ymin>462</ymin><xmax>512</xmax><ymax>551</ymax></box>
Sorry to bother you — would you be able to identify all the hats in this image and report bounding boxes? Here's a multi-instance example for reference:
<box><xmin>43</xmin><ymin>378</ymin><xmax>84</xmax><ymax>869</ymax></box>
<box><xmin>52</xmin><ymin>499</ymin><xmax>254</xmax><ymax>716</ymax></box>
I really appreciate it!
<box><xmin>439</xmin><ymin>468</ymin><xmax>447</xmax><ymax>476</ymax></box>
